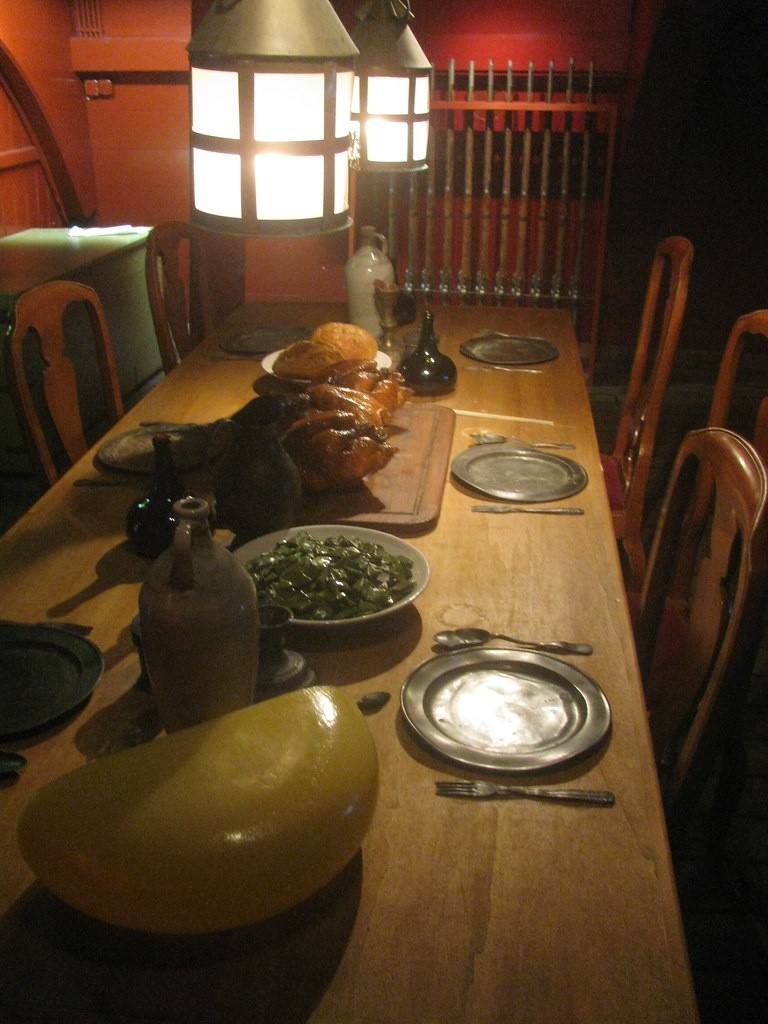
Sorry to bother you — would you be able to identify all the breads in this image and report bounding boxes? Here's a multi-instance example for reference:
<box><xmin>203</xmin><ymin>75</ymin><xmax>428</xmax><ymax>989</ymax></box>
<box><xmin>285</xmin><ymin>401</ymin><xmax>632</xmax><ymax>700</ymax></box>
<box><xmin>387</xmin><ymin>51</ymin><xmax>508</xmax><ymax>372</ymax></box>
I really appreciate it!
<box><xmin>308</xmin><ymin>322</ymin><xmax>379</xmax><ymax>360</ymax></box>
<box><xmin>271</xmin><ymin>339</ymin><xmax>347</xmax><ymax>381</ymax></box>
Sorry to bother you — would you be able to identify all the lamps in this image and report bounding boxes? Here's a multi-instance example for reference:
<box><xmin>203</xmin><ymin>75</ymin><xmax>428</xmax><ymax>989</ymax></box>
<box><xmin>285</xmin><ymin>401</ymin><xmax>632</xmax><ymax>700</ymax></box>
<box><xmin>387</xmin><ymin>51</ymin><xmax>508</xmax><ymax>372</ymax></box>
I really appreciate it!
<box><xmin>186</xmin><ymin>0</ymin><xmax>360</xmax><ymax>240</ymax></box>
<box><xmin>348</xmin><ymin>0</ymin><xmax>434</xmax><ymax>176</ymax></box>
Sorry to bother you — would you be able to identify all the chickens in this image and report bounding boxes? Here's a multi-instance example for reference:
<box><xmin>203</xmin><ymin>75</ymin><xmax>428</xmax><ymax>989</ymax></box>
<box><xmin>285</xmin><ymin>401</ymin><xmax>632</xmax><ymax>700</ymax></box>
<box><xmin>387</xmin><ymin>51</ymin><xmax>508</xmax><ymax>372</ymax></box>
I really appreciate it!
<box><xmin>211</xmin><ymin>358</ymin><xmax>416</xmax><ymax>490</ymax></box>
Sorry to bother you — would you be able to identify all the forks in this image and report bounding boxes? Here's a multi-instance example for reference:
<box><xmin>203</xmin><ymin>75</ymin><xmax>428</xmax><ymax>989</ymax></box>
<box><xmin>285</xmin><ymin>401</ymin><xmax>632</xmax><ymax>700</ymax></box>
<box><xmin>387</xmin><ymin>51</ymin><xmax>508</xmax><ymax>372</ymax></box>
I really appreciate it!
<box><xmin>471</xmin><ymin>504</ymin><xmax>585</xmax><ymax>515</ymax></box>
<box><xmin>434</xmin><ymin>780</ymin><xmax>616</xmax><ymax>806</ymax></box>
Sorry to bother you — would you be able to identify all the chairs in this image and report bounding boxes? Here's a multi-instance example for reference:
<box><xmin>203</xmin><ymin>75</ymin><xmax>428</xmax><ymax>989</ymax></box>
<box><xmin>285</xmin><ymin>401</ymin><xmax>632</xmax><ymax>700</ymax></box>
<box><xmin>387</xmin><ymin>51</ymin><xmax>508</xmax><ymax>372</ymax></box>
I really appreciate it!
<box><xmin>600</xmin><ymin>235</ymin><xmax>768</xmax><ymax>816</ymax></box>
<box><xmin>145</xmin><ymin>221</ymin><xmax>217</xmax><ymax>376</ymax></box>
<box><xmin>0</xmin><ymin>279</ymin><xmax>124</xmax><ymax>490</ymax></box>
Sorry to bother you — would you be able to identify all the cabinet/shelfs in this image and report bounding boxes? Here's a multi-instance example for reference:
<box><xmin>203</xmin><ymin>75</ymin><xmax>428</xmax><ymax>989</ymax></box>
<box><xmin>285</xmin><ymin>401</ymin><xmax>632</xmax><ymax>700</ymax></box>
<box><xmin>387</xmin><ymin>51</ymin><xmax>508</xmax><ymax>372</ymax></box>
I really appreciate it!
<box><xmin>0</xmin><ymin>226</ymin><xmax>162</xmax><ymax>480</ymax></box>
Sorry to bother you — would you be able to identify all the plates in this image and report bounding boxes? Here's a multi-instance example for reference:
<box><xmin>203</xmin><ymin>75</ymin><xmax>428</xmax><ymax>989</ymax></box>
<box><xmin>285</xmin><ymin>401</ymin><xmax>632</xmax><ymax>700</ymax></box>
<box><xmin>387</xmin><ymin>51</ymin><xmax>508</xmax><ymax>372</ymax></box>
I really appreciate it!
<box><xmin>218</xmin><ymin>325</ymin><xmax>312</xmax><ymax>355</ymax></box>
<box><xmin>400</xmin><ymin>645</ymin><xmax>612</xmax><ymax>775</ymax></box>
<box><xmin>459</xmin><ymin>334</ymin><xmax>560</xmax><ymax>365</ymax></box>
<box><xmin>261</xmin><ymin>346</ymin><xmax>393</xmax><ymax>385</ymax></box>
<box><xmin>232</xmin><ymin>523</ymin><xmax>430</xmax><ymax>629</ymax></box>
<box><xmin>0</xmin><ymin>622</ymin><xmax>107</xmax><ymax>736</ymax></box>
<box><xmin>96</xmin><ymin>422</ymin><xmax>203</xmax><ymax>471</ymax></box>
<box><xmin>451</xmin><ymin>443</ymin><xmax>588</xmax><ymax>503</ymax></box>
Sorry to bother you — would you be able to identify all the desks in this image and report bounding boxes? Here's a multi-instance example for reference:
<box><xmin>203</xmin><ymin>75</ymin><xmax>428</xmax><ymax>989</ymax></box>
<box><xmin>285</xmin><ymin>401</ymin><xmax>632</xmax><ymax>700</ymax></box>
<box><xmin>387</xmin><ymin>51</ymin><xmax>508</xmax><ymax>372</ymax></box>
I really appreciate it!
<box><xmin>0</xmin><ymin>301</ymin><xmax>701</xmax><ymax>1024</ymax></box>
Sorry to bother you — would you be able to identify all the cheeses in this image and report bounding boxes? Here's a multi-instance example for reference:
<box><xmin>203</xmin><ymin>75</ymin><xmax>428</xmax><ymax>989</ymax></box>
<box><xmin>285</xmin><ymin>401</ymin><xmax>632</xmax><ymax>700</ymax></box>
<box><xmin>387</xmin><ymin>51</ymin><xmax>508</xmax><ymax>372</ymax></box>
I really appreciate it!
<box><xmin>14</xmin><ymin>684</ymin><xmax>379</xmax><ymax>934</ymax></box>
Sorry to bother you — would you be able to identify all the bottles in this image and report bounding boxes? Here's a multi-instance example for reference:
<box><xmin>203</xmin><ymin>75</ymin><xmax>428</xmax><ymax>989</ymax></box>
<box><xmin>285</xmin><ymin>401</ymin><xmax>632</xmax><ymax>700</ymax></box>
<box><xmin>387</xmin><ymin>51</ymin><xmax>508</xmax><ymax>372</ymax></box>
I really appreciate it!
<box><xmin>344</xmin><ymin>224</ymin><xmax>395</xmax><ymax>338</ymax></box>
<box><xmin>138</xmin><ymin>496</ymin><xmax>266</xmax><ymax>735</ymax></box>
<box><xmin>397</xmin><ymin>309</ymin><xmax>458</xmax><ymax>396</ymax></box>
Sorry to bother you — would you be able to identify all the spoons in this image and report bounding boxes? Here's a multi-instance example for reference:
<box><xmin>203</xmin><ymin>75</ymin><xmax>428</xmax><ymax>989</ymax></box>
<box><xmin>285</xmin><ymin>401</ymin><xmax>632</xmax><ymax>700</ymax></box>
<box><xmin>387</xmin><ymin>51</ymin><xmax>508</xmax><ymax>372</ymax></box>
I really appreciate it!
<box><xmin>431</xmin><ymin>627</ymin><xmax>594</xmax><ymax>656</ymax></box>
<box><xmin>469</xmin><ymin>433</ymin><xmax>576</xmax><ymax>450</ymax></box>
